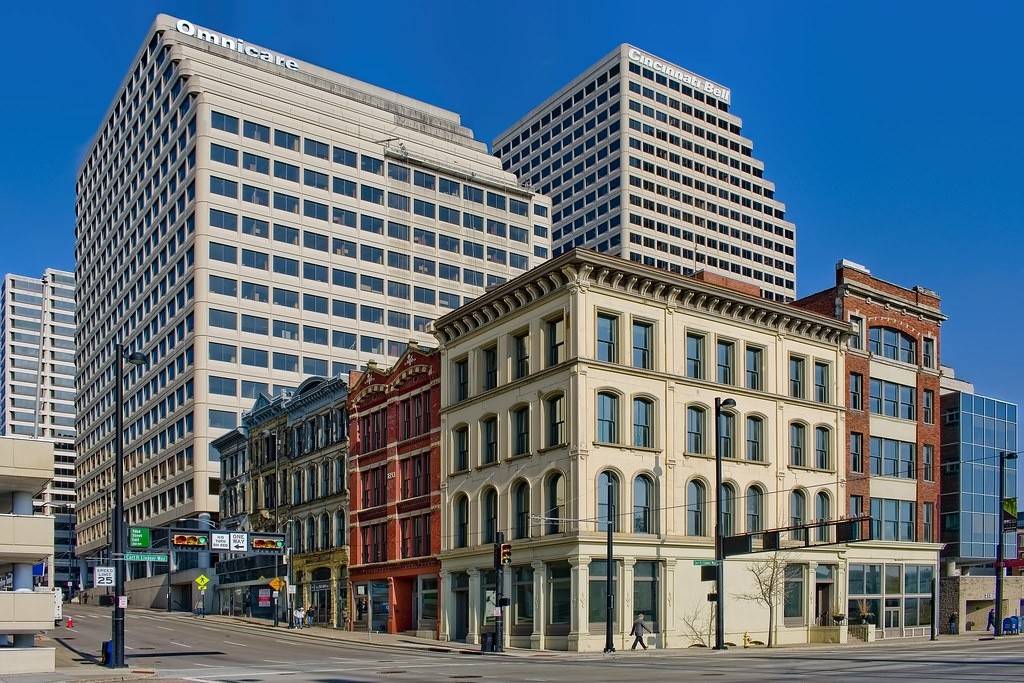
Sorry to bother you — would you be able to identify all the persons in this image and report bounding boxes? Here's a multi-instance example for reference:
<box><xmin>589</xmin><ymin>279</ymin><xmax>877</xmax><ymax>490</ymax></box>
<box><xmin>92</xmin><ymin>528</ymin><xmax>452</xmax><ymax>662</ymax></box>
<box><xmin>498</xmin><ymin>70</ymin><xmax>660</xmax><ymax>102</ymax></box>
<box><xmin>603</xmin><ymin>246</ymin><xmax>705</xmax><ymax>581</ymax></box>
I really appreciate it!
<box><xmin>948</xmin><ymin>612</ymin><xmax>958</xmax><ymax>635</ymax></box>
<box><xmin>195</xmin><ymin>599</ymin><xmax>203</xmax><ymax>616</ymax></box>
<box><xmin>342</xmin><ymin>607</ymin><xmax>348</xmax><ymax>630</ymax></box>
<box><xmin>83</xmin><ymin>593</ymin><xmax>88</xmax><ymax>604</ymax></box>
<box><xmin>126</xmin><ymin>593</ymin><xmax>131</xmax><ymax>606</ymax></box>
<box><xmin>294</xmin><ymin>605</ymin><xmax>315</xmax><ymax>630</ymax></box>
<box><xmin>986</xmin><ymin>609</ymin><xmax>995</xmax><ymax>631</ymax></box>
<box><xmin>629</xmin><ymin>614</ymin><xmax>652</xmax><ymax>651</ymax></box>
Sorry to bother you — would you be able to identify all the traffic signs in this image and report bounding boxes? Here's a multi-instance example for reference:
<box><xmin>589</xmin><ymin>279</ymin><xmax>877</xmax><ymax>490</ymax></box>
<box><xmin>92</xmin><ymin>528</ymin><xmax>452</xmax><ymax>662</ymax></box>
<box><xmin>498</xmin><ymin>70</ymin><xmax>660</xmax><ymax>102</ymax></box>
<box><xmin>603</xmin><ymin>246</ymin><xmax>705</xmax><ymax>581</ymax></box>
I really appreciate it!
<box><xmin>230</xmin><ymin>533</ymin><xmax>247</xmax><ymax>552</ymax></box>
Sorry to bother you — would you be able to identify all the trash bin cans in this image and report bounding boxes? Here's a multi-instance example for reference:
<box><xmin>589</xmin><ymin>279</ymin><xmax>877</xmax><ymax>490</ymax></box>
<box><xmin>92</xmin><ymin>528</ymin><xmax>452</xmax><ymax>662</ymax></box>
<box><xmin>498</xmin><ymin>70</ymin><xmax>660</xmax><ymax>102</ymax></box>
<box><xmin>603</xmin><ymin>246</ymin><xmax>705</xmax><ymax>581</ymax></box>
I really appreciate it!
<box><xmin>1011</xmin><ymin>615</ymin><xmax>1024</xmax><ymax>634</ymax></box>
<box><xmin>99</xmin><ymin>595</ymin><xmax>113</xmax><ymax>606</ymax></box>
<box><xmin>102</xmin><ymin>640</ymin><xmax>112</xmax><ymax>666</ymax></box>
<box><xmin>1003</xmin><ymin>618</ymin><xmax>1017</xmax><ymax>635</ymax></box>
<box><xmin>481</xmin><ymin>632</ymin><xmax>495</xmax><ymax>652</ymax></box>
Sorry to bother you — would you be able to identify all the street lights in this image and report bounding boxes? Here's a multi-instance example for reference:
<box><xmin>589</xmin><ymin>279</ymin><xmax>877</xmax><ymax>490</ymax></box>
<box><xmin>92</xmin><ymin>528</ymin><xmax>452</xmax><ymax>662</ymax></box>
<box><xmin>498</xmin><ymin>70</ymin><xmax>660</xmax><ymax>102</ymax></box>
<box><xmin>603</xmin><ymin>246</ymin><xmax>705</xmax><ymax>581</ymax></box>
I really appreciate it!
<box><xmin>62</xmin><ymin>504</ymin><xmax>72</xmax><ymax>603</ymax></box>
<box><xmin>261</xmin><ymin>429</ymin><xmax>279</xmax><ymax>627</ymax></box>
<box><xmin>113</xmin><ymin>343</ymin><xmax>148</xmax><ymax>667</ymax></box>
<box><xmin>98</xmin><ymin>489</ymin><xmax>108</xmax><ymax>595</ymax></box>
<box><xmin>995</xmin><ymin>451</ymin><xmax>1018</xmax><ymax>636</ymax></box>
<box><xmin>715</xmin><ymin>396</ymin><xmax>736</xmax><ymax>648</ymax></box>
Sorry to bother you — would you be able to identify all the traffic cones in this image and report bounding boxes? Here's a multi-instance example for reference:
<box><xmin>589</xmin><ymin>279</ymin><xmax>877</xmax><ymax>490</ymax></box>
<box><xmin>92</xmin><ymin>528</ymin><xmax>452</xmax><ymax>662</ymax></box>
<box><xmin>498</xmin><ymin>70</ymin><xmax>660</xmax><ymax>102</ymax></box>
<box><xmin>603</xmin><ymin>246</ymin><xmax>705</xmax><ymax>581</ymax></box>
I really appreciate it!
<box><xmin>66</xmin><ymin>615</ymin><xmax>73</xmax><ymax>628</ymax></box>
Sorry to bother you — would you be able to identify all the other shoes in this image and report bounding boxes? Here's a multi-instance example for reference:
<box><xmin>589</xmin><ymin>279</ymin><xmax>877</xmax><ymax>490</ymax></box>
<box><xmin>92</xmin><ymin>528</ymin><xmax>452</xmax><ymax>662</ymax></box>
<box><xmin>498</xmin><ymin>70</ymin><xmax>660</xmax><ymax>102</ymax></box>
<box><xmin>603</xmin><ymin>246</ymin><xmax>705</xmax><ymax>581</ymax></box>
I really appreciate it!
<box><xmin>632</xmin><ymin>649</ymin><xmax>634</xmax><ymax>650</ymax></box>
<box><xmin>645</xmin><ymin>646</ymin><xmax>648</xmax><ymax>649</ymax></box>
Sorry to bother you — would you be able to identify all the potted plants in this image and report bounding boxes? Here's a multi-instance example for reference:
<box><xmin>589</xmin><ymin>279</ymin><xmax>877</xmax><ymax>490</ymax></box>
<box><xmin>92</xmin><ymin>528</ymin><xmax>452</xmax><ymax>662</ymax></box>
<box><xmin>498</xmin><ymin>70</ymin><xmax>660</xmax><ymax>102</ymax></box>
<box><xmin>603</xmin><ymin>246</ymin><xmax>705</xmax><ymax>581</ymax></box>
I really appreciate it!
<box><xmin>832</xmin><ymin>613</ymin><xmax>845</xmax><ymax>625</ymax></box>
<box><xmin>860</xmin><ymin>612</ymin><xmax>875</xmax><ymax>624</ymax></box>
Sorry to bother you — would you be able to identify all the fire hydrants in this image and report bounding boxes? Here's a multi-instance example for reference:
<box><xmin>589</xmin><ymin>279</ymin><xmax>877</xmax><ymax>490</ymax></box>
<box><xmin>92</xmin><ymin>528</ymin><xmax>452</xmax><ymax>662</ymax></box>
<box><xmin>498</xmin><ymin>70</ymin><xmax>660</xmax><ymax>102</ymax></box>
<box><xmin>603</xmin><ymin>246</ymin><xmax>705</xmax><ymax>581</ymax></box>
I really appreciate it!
<box><xmin>743</xmin><ymin>632</ymin><xmax>751</xmax><ymax>648</ymax></box>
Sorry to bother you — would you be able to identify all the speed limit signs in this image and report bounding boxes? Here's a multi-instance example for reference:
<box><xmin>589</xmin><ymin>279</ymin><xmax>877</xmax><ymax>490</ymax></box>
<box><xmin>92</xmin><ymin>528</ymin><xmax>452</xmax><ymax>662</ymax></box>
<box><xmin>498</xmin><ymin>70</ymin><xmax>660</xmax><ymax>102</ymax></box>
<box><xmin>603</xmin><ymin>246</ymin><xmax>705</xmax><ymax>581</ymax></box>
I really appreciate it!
<box><xmin>94</xmin><ymin>566</ymin><xmax>116</xmax><ymax>587</ymax></box>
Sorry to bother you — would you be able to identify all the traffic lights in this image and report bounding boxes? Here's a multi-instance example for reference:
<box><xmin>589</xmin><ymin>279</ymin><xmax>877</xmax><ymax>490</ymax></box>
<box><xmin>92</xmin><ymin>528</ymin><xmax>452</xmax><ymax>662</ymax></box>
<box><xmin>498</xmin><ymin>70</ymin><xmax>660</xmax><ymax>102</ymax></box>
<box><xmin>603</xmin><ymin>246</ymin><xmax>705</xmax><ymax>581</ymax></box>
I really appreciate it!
<box><xmin>174</xmin><ymin>535</ymin><xmax>209</xmax><ymax>546</ymax></box>
<box><xmin>501</xmin><ymin>544</ymin><xmax>511</xmax><ymax>566</ymax></box>
<box><xmin>253</xmin><ymin>538</ymin><xmax>285</xmax><ymax>549</ymax></box>
<box><xmin>499</xmin><ymin>597</ymin><xmax>510</xmax><ymax>606</ymax></box>
